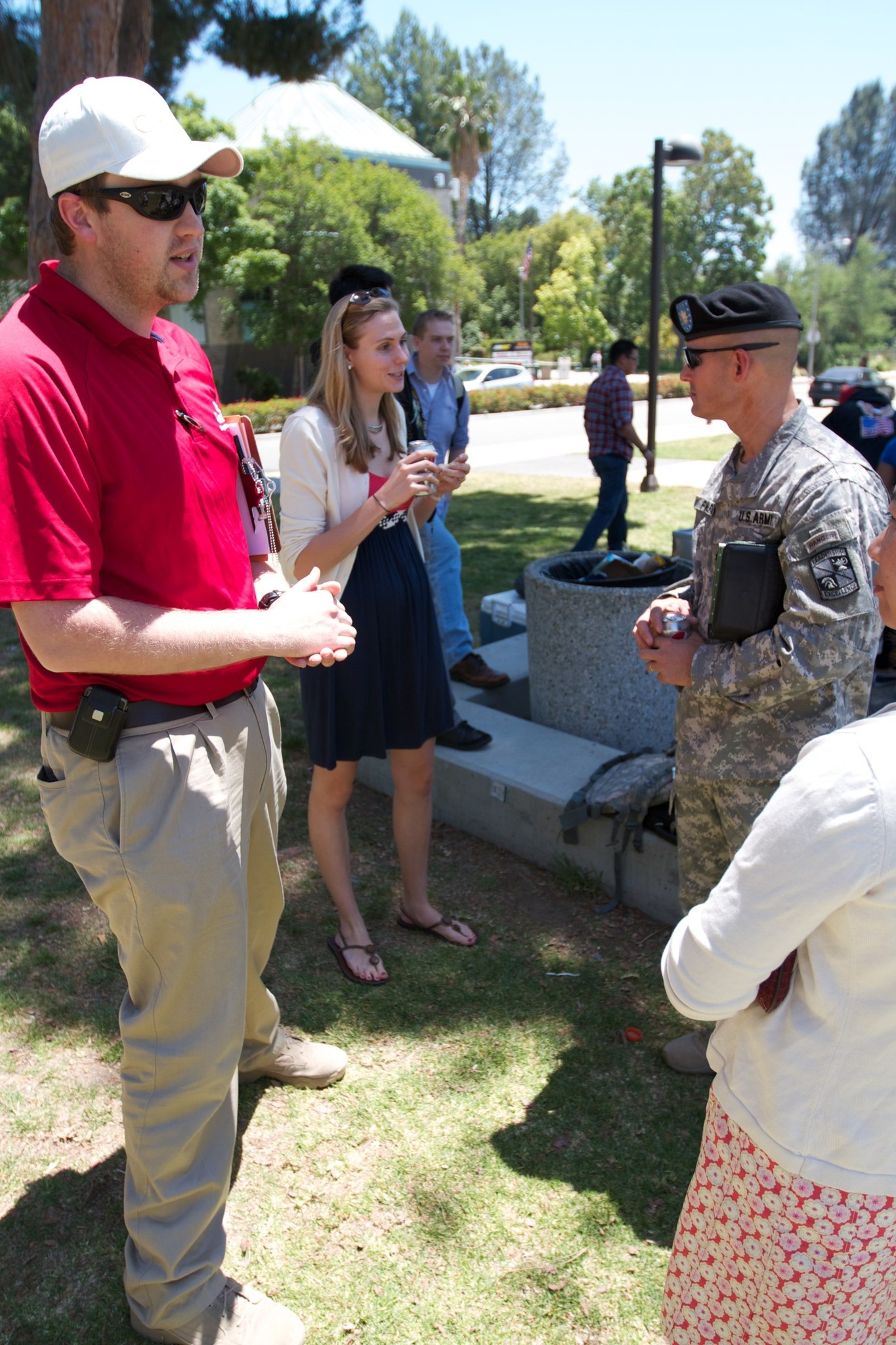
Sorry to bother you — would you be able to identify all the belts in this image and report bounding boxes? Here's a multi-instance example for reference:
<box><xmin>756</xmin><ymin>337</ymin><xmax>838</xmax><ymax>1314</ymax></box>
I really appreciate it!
<box><xmin>45</xmin><ymin>675</ymin><xmax>259</xmax><ymax>731</ymax></box>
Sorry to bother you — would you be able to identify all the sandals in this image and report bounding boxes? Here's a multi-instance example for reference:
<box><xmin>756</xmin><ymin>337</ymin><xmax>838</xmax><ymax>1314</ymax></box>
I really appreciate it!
<box><xmin>396</xmin><ymin>903</ymin><xmax>478</xmax><ymax>946</ymax></box>
<box><xmin>327</xmin><ymin>928</ymin><xmax>390</xmax><ymax>985</ymax></box>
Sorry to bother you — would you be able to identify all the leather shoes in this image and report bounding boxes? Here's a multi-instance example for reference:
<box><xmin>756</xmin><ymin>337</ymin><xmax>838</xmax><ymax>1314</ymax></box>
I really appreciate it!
<box><xmin>435</xmin><ymin>720</ymin><xmax>492</xmax><ymax>751</ymax></box>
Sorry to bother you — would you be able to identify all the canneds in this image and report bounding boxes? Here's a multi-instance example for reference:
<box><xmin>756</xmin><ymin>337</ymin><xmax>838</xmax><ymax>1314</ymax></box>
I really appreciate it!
<box><xmin>408</xmin><ymin>440</ymin><xmax>437</xmax><ymax>496</ymax></box>
<box><xmin>651</xmin><ymin>612</ymin><xmax>691</xmax><ymax>639</ymax></box>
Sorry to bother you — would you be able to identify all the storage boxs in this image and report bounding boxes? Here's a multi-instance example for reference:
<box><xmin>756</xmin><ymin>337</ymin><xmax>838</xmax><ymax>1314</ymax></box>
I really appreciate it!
<box><xmin>479</xmin><ymin>589</ymin><xmax>527</xmax><ymax>645</ymax></box>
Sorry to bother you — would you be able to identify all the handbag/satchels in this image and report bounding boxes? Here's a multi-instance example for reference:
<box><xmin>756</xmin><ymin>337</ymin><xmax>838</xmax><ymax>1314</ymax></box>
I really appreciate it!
<box><xmin>707</xmin><ymin>540</ymin><xmax>787</xmax><ymax>642</ymax></box>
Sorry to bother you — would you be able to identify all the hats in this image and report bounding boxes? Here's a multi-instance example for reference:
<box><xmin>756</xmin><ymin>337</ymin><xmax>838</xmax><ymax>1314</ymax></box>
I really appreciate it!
<box><xmin>670</xmin><ymin>282</ymin><xmax>804</xmax><ymax>342</ymax></box>
<box><xmin>38</xmin><ymin>76</ymin><xmax>244</xmax><ymax>200</ymax></box>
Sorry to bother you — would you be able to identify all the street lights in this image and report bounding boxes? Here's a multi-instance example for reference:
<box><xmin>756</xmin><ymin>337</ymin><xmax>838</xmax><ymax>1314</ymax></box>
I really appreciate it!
<box><xmin>641</xmin><ymin>137</ymin><xmax>701</xmax><ymax>494</ymax></box>
<box><xmin>807</xmin><ymin>238</ymin><xmax>851</xmax><ymax>378</ymax></box>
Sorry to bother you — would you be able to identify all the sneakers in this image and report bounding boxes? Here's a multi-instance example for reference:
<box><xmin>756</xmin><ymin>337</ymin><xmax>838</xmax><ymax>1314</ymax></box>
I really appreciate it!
<box><xmin>238</xmin><ymin>1032</ymin><xmax>347</xmax><ymax>1089</ymax></box>
<box><xmin>662</xmin><ymin>1026</ymin><xmax>715</xmax><ymax>1074</ymax></box>
<box><xmin>129</xmin><ymin>1276</ymin><xmax>306</xmax><ymax>1345</ymax></box>
<box><xmin>449</xmin><ymin>651</ymin><xmax>511</xmax><ymax>690</ymax></box>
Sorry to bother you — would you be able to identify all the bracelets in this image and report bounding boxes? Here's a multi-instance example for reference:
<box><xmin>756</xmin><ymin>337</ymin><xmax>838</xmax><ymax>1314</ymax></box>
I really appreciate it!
<box><xmin>257</xmin><ymin>590</ymin><xmax>285</xmax><ymax>611</ymax></box>
<box><xmin>373</xmin><ymin>494</ymin><xmax>390</xmax><ymax>512</ymax></box>
<box><xmin>431</xmin><ymin>495</ymin><xmax>441</xmax><ymax>502</ymax></box>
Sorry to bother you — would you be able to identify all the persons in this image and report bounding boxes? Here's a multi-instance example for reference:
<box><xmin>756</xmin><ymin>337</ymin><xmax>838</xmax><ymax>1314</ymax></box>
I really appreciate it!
<box><xmin>570</xmin><ymin>339</ymin><xmax>654</xmax><ymax>552</ymax></box>
<box><xmin>0</xmin><ymin>74</ymin><xmax>356</xmax><ymax>1345</ymax></box>
<box><xmin>658</xmin><ymin>477</ymin><xmax>896</xmax><ymax>1345</ymax></box>
<box><xmin>281</xmin><ymin>265</ymin><xmax>511</xmax><ymax>986</ymax></box>
<box><xmin>589</xmin><ymin>348</ymin><xmax>602</xmax><ymax>378</ymax></box>
<box><xmin>630</xmin><ymin>283</ymin><xmax>896</xmax><ymax>1076</ymax></box>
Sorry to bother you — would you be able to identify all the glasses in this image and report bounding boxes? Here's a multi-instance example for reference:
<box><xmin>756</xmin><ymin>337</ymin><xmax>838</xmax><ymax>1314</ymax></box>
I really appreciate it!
<box><xmin>682</xmin><ymin>342</ymin><xmax>780</xmax><ymax>368</ymax></box>
<box><xmin>345</xmin><ymin>287</ymin><xmax>389</xmax><ymax>319</ymax></box>
<box><xmin>627</xmin><ymin>355</ymin><xmax>638</xmax><ymax>362</ymax></box>
<box><xmin>73</xmin><ymin>178</ymin><xmax>207</xmax><ymax>221</ymax></box>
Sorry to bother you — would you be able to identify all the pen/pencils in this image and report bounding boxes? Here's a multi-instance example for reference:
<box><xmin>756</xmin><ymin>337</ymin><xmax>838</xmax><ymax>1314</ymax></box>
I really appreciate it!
<box><xmin>175</xmin><ymin>410</ymin><xmax>206</xmax><ymax>433</ymax></box>
<box><xmin>233</xmin><ymin>435</ymin><xmax>262</xmax><ymax>515</ymax></box>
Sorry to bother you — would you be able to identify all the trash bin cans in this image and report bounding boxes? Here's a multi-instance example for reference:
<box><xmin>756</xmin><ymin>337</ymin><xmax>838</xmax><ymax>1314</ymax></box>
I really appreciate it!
<box><xmin>523</xmin><ymin>551</ymin><xmax>691</xmax><ymax>755</ymax></box>
<box><xmin>539</xmin><ymin>365</ymin><xmax>551</xmax><ymax>380</ymax></box>
<box><xmin>558</xmin><ymin>356</ymin><xmax>571</xmax><ymax>378</ymax></box>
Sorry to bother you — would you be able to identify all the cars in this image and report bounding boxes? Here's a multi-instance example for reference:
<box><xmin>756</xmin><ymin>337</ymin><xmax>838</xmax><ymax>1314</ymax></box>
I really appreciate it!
<box><xmin>809</xmin><ymin>366</ymin><xmax>895</xmax><ymax>407</ymax></box>
<box><xmin>455</xmin><ymin>363</ymin><xmax>535</xmax><ymax>392</ymax></box>
